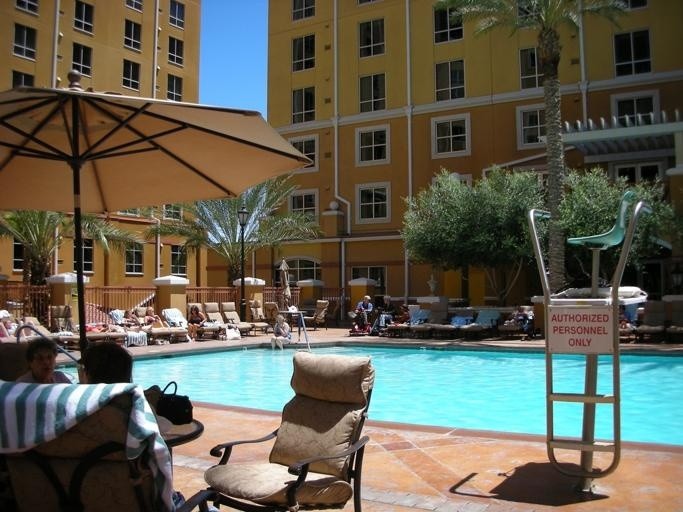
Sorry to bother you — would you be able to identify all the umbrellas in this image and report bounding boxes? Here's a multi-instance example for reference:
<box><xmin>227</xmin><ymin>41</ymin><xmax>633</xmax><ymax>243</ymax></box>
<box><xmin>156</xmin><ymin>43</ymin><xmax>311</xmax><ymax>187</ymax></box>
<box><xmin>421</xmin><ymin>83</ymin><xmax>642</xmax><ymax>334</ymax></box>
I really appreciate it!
<box><xmin>0</xmin><ymin>69</ymin><xmax>314</xmax><ymax>346</ymax></box>
<box><xmin>276</xmin><ymin>256</ymin><xmax>292</xmax><ymax>310</ymax></box>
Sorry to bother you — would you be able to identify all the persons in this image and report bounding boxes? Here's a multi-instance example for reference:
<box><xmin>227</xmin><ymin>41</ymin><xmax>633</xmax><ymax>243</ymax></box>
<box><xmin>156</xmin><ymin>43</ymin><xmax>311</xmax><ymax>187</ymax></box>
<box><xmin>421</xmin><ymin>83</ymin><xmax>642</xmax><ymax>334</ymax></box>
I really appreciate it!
<box><xmin>355</xmin><ymin>295</ymin><xmax>410</xmax><ymax>337</ymax></box>
<box><xmin>187</xmin><ymin>305</ymin><xmax>206</xmax><ymax>340</ymax></box>
<box><xmin>76</xmin><ymin>341</ymin><xmax>172</xmax><ymax>438</ymax></box>
<box><xmin>15</xmin><ymin>336</ymin><xmax>72</xmax><ymax>384</ymax></box>
<box><xmin>503</xmin><ymin>307</ymin><xmax>527</xmax><ymax>327</ymax></box>
<box><xmin>270</xmin><ymin>314</ymin><xmax>292</xmax><ymax>350</ymax></box>
<box><xmin>144</xmin><ymin>306</ymin><xmax>170</xmax><ymax>328</ymax></box>
<box><xmin>0</xmin><ymin>309</ymin><xmax>34</xmax><ymax>338</ymax></box>
<box><xmin>121</xmin><ymin>309</ymin><xmax>153</xmax><ymax>333</ymax></box>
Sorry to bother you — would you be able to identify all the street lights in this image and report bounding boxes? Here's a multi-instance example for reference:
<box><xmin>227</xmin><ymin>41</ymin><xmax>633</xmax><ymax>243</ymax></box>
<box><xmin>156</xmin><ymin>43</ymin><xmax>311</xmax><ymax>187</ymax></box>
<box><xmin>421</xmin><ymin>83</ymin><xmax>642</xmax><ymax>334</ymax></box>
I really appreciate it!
<box><xmin>235</xmin><ymin>203</ymin><xmax>250</xmax><ymax>318</ymax></box>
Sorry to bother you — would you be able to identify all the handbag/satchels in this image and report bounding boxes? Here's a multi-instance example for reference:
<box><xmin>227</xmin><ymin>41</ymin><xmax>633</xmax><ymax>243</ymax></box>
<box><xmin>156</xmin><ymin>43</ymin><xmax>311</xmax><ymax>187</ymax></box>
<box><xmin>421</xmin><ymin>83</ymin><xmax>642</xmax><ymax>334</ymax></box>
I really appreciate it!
<box><xmin>155</xmin><ymin>380</ymin><xmax>193</xmax><ymax>426</ymax></box>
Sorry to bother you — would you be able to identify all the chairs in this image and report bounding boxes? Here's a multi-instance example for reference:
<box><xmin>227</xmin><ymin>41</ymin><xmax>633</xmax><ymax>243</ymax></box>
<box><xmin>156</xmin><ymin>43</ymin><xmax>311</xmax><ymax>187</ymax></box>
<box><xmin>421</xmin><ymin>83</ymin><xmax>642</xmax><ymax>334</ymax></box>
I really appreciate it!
<box><xmin>665</xmin><ymin>300</ymin><xmax>683</xmax><ymax>344</ymax></box>
<box><xmin>303</xmin><ymin>300</ymin><xmax>329</xmax><ymax>331</ymax></box>
<box><xmin>349</xmin><ymin>295</ymin><xmax>533</xmax><ymax>341</ymax></box>
<box><xmin>0</xmin><ymin>342</ymin><xmax>36</xmax><ymax>381</ymax></box>
<box><xmin>568</xmin><ymin>191</ymin><xmax>633</xmax><ymax>297</ymax></box>
<box><xmin>176</xmin><ymin>351</ymin><xmax>375</xmax><ymax>512</ymax></box>
<box><xmin>636</xmin><ymin>300</ymin><xmax>666</xmax><ymax>343</ymax></box>
<box><xmin>619</xmin><ymin>303</ymin><xmax>638</xmax><ymax>344</ymax></box>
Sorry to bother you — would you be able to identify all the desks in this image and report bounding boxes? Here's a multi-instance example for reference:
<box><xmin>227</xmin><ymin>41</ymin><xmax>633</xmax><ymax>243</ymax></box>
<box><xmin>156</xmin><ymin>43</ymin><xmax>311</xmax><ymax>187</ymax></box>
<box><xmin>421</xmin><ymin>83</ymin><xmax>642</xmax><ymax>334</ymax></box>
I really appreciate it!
<box><xmin>156</xmin><ymin>419</ymin><xmax>204</xmax><ymax>482</ymax></box>
